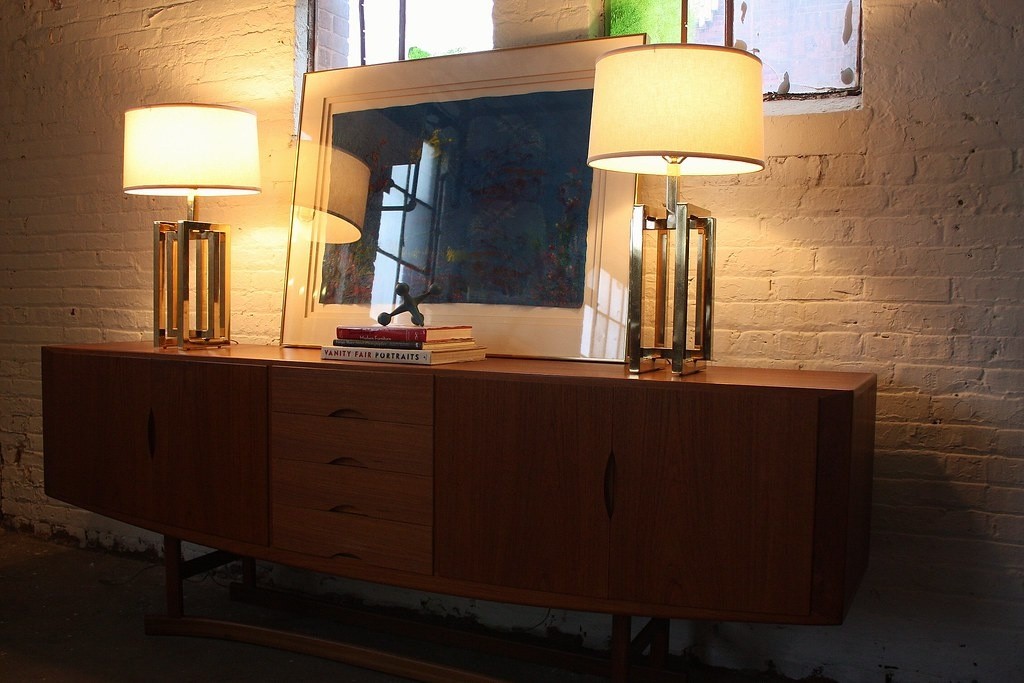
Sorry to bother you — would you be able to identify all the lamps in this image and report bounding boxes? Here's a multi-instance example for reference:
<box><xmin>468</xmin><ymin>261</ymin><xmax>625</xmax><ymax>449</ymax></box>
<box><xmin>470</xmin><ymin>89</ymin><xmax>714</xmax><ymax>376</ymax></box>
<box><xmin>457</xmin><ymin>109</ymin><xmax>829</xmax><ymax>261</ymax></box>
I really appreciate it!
<box><xmin>121</xmin><ymin>103</ymin><xmax>262</xmax><ymax>349</ymax></box>
<box><xmin>324</xmin><ymin>144</ymin><xmax>371</xmax><ymax>245</ymax></box>
<box><xmin>586</xmin><ymin>43</ymin><xmax>766</xmax><ymax>378</ymax></box>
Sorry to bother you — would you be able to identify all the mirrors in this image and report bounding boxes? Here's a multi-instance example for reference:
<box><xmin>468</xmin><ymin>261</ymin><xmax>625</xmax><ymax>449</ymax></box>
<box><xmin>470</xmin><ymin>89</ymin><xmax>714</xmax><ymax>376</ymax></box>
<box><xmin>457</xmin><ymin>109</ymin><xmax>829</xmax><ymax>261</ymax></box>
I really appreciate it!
<box><xmin>278</xmin><ymin>31</ymin><xmax>650</xmax><ymax>364</ymax></box>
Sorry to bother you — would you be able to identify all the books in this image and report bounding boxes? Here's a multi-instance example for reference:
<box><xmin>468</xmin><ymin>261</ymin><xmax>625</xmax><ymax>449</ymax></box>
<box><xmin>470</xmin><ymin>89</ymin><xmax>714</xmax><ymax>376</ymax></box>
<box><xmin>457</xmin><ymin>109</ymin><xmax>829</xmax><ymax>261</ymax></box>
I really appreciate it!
<box><xmin>322</xmin><ymin>326</ymin><xmax>488</xmax><ymax>365</ymax></box>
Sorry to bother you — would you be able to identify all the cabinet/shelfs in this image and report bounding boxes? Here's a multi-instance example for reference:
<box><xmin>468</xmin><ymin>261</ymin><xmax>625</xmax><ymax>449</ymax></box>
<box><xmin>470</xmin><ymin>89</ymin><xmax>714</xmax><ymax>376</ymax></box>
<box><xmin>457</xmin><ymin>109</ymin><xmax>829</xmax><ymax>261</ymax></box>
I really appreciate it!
<box><xmin>431</xmin><ymin>354</ymin><xmax>879</xmax><ymax>682</ymax></box>
<box><xmin>40</xmin><ymin>341</ymin><xmax>264</xmax><ymax>644</ymax></box>
<box><xmin>264</xmin><ymin>348</ymin><xmax>433</xmax><ymax>680</ymax></box>
